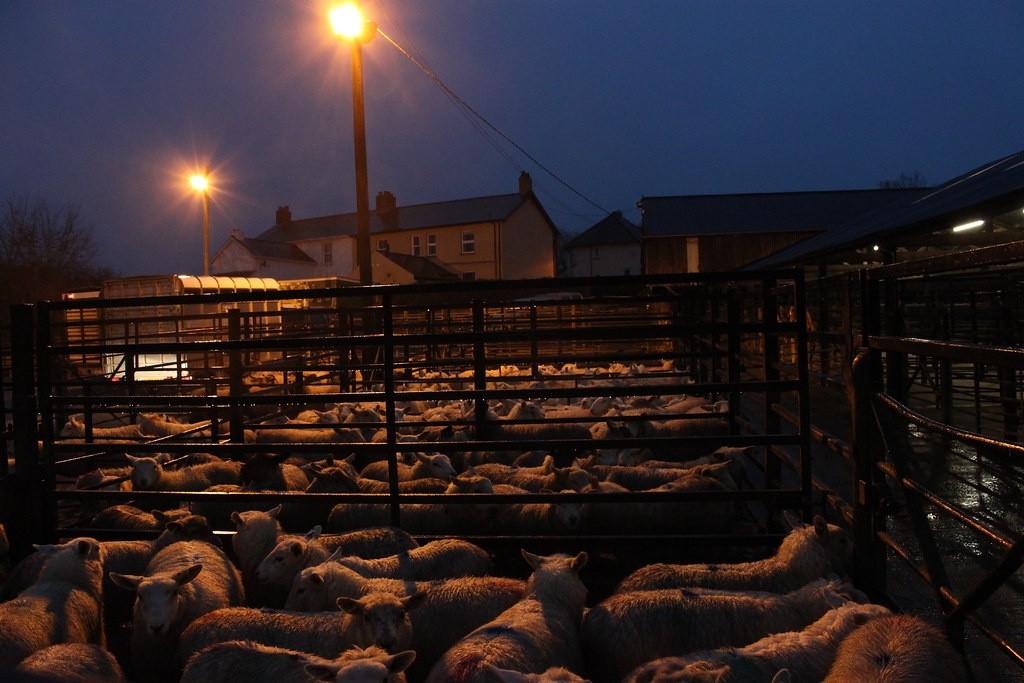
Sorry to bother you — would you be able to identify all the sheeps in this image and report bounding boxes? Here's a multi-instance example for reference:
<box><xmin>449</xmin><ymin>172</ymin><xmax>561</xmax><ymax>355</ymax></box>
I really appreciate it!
<box><xmin>0</xmin><ymin>357</ymin><xmax>966</xmax><ymax>683</ymax></box>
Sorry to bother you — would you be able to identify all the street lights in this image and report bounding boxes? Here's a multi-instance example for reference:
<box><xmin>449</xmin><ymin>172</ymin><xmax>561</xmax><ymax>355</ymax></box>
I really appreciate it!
<box><xmin>322</xmin><ymin>0</ymin><xmax>376</xmax><ymax>370</ymax></box>
<box><xmin>189</xmin><ymin>164</ymin><xmax>209</xmax><ymax>276</ymax></box>
